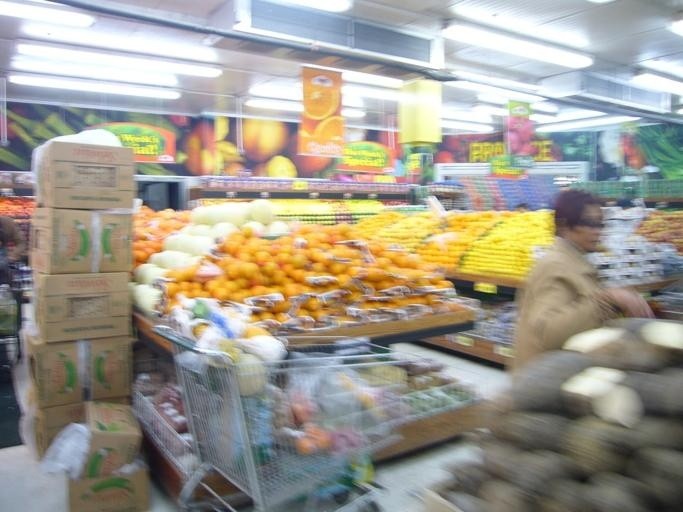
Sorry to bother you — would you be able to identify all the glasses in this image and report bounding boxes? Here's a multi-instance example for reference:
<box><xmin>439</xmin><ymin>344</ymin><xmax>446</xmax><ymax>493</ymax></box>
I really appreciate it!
<box><xmin>577</xmin><ymin>218</ymin><xmax>605</xmax><ymax>229</ymax></box>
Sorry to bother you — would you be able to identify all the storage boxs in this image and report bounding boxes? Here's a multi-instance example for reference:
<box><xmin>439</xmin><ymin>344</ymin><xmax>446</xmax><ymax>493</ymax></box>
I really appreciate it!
<box><xmin>28</xmin><ymin>143</ymin><xmax>135</xmax><ymax>343</ymax></box>
<box><xmin>19</xmin><ymin>330</ymin><xmax>143</xmax><ymax>474</ymax></box>
<box><xmin>63</xmin><ymin>461</ymin><xmax>149</xmax><ymax>508</ymax></box>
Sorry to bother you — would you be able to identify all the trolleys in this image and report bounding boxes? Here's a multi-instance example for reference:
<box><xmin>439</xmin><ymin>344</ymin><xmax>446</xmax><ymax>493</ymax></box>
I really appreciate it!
<box><xmin>147</xmin><ymin>325</ymin><xmax>421</xmax><ymax>512</ymax></box>
<box><xmin>0</xmin><ymin>267</ymin><xmax>21</xmax><ymax>376</ymax></box>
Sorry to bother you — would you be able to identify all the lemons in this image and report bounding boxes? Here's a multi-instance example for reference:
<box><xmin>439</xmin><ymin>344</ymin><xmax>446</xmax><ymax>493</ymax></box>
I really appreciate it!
<box><xmin>359</xmin><ymin>208</ymin><xmax>554</xmax><ymax>280</ymax></box>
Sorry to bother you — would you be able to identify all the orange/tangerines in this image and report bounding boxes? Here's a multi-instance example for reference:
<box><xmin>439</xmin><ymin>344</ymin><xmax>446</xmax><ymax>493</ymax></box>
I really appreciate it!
<box><xmin>132</xmin><ymin>198</ymin><xmax>455</xmax><ymax>336</ymax></box>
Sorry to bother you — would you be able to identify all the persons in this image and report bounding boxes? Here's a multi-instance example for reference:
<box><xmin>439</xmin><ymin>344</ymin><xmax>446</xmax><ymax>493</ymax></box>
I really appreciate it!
<box><xmin>508</xmin><ymin>190</ymin><xmax>658</xmax><ymax>367</ymax></box>
<box><xmin>0</xmin><ymin>216</ymin><xmax>26</xmax><ymax>283</ymax></box>
